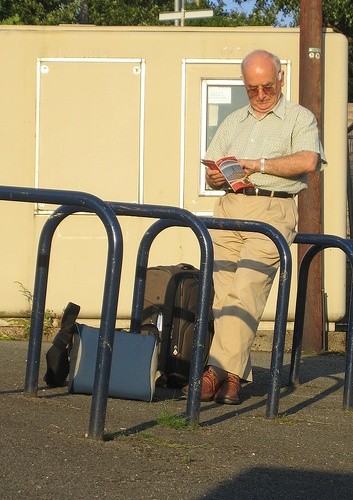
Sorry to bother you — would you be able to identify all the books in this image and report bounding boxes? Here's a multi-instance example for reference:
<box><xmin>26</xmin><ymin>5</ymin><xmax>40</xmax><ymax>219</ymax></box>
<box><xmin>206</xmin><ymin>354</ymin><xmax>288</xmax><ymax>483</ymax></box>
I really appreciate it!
<box><xmin>201</xmin><ymin>156</ymin><xmax>254</xmax><ymax>192</ymax></box>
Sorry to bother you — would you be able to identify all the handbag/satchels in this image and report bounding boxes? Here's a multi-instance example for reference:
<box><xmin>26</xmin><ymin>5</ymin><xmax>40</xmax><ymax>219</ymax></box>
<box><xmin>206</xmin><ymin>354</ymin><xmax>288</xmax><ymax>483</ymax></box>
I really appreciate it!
<box><xmin>68</xmin><ymin>322</ymin><xmax>161</xmax><ymax>402</ymax></box>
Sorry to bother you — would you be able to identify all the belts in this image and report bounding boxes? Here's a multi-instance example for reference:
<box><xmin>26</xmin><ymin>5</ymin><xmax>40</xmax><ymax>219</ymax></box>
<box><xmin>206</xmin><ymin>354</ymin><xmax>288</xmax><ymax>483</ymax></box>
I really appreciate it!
<box><xmin>224</xmin><ymin>187</ymin><xmax>296</xmax><ymax>198</ymax></box>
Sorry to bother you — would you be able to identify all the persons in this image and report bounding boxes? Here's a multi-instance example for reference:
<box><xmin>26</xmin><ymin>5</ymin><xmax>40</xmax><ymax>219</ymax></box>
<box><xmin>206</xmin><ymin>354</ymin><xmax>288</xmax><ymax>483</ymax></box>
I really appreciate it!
<box><xmin>181</xmin><ymin>49</ymin><xmax>327</xmax><ymax>405</ymax></box>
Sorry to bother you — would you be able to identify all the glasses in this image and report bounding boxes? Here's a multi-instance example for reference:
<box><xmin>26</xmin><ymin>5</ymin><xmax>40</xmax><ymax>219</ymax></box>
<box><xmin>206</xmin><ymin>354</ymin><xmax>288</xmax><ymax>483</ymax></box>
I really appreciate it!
<box><xmin>246</xmin><ymin>79</ymin><xmax>277</xmax><ymax>96</ymax></box>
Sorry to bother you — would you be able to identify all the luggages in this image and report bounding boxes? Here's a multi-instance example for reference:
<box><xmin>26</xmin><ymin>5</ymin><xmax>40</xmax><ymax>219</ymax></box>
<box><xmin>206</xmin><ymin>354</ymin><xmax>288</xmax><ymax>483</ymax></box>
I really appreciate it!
<box><xmin>140</xmin><ymin>263</ymin><xmax>215</xmax><ymax>389</ymax></box>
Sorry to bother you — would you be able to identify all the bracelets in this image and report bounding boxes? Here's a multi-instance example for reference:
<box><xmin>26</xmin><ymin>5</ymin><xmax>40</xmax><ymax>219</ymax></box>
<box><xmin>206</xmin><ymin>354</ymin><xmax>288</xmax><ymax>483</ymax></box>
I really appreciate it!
<box><xmin>260</xmin><ymin>158</ymin><xmax>265</xmax><ymax>174</ymax></box>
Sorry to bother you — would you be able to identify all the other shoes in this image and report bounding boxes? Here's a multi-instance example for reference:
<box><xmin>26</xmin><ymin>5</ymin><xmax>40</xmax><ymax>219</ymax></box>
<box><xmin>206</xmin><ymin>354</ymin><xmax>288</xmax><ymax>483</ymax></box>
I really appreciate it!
<box><xmin>182</xmin><ymin>369</ymin><xmax>224</xmax><ymax>402</ymax></box>
<box><xmin>213</xmin><ymin>373</ymin><xmax>242</xmax><ymax>404</ymax></box>
